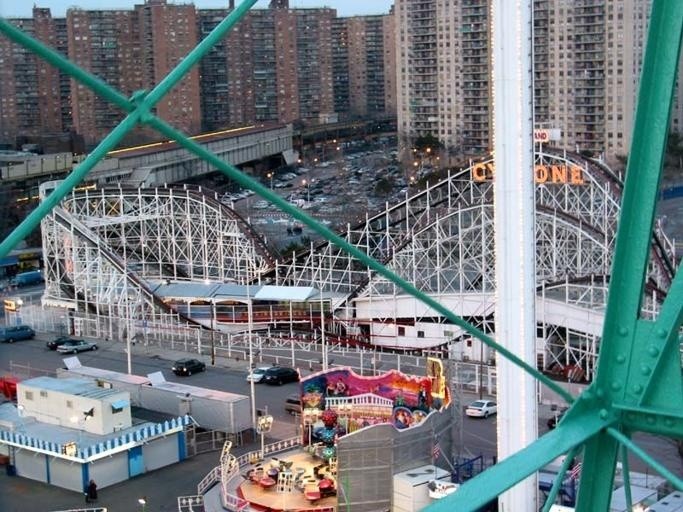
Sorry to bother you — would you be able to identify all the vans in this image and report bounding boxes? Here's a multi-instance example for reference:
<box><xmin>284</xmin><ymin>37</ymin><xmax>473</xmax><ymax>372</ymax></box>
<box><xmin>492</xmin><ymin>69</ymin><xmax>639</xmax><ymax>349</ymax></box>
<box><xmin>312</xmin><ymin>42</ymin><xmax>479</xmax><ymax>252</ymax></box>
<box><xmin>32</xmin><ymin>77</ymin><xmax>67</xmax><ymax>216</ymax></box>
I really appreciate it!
<box><xmin>285</xmin><ymin>393</ymin><xmax>301</xmax><ymax>415</ymax></box>
<box><xmin>0</xmin><ymin>325</ymin><xmax>36</xmax><ymax>344</ymax></box>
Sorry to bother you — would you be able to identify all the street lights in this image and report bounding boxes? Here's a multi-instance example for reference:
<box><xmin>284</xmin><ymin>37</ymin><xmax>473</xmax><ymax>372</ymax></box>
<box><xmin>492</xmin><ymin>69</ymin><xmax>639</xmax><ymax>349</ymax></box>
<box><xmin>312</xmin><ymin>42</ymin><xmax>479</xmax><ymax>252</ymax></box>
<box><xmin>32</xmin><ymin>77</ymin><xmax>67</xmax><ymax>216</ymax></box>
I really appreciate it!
<box><xmin>4</xmin><ymin>152</ymin><xmax>85</xmax><ymax>180</ymax></box>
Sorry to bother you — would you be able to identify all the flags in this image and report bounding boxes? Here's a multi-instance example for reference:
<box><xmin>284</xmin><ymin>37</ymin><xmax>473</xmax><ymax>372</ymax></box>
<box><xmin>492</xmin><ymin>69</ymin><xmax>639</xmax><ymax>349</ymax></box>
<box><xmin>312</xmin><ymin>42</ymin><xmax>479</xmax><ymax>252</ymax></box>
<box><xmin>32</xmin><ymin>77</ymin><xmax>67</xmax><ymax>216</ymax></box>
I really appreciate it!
<box><xmin>568</xmin><ymin>366</ymin><xmax>586</xmax><ymax>385</ymax></box>
<box><xmin>432</xmin><ymin>438</ymin><xmax>440</xmax><ymax>460</ymax></box>
<box><xmin>570</xmin><ymin>461</ymin><xmax>581</xmax><ymax>480</ymax></box>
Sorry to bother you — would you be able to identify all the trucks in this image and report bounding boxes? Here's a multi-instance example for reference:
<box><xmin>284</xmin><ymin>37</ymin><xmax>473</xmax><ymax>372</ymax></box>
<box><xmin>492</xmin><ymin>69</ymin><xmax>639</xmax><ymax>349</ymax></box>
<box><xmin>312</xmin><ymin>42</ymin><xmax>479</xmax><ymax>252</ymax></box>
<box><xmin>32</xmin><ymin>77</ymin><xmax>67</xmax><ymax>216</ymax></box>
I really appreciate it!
<box><xmin>11</xmin><ymin>270</ymin><xmax>43</xmax><ymax>287</ymax></box>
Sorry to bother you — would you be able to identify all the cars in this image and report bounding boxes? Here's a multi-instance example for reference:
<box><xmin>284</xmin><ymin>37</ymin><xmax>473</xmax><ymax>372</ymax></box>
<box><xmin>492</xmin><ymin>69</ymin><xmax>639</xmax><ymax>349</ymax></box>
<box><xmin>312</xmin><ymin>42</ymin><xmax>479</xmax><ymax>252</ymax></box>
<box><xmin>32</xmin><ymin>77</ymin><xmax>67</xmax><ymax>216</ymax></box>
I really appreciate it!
<box><xmin>220</xmin><ymin>142</ymin><xmax>438</xmax><ymax>235</ymax></box>
<box><xmin>57</xmin><ymin>339</ymin><xmax>98</xmax><ymax>354</ymax></box>
<box><xmin>246</xmin><ymin>367</ymin><xmax>272</xmax><ymax>384</ymax></box>
<box><xmin>465</xmin><ymin>398</ymin><xmax>497</xmax><ymax>419</ymax></box>
<box><xmin>46</xmin><ymin>337</ymin><xmax>84</xmax><ymax>349</ymax></box>
<box><xmin>548</xmin><ymin>415</ymin><xmax>563</xmax><ymax>429</ymax></box>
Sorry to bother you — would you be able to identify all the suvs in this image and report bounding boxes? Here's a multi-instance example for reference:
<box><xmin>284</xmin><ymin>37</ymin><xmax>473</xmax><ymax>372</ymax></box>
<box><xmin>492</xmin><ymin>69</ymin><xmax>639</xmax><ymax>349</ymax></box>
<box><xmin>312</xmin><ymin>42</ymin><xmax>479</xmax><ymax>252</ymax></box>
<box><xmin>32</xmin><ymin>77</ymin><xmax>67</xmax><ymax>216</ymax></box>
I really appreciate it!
<box><xmin>171</xmin><ymin>358</ymin><xmax>206</xmax><ymax>377</ymax></box>
<box><xmin>263</xmin><ymin>366</ymin><xmax>299</xmax><ymax>385</ymax></box>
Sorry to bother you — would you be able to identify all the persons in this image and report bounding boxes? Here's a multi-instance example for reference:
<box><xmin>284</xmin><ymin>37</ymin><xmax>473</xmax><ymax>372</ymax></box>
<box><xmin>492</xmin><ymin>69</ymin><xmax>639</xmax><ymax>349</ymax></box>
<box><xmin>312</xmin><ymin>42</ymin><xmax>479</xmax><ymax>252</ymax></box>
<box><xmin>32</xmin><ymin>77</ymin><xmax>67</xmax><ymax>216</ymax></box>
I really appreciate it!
<box><xmin>335</xmin><ymin>377</ymin><xmax>345</xmax><ymax>404</ymax></box>
<box><xmin>416</xmin><ymin>385</ymin><xmax>428</xmax><ymax>410</ymax></box>
<box><xmin>84</xmin><ymin>479</ymin><xmax>97</xmax><ymax>505</ymax></box>
<box><xmin>326</xmin><ymin>381</ymin><xmax>335</xmax><ymax>404</ymax></box>
<box><xmin>342</xmin><ymin>383</ymin><xmax>351</xmax><ymax>404</ymax></box>
